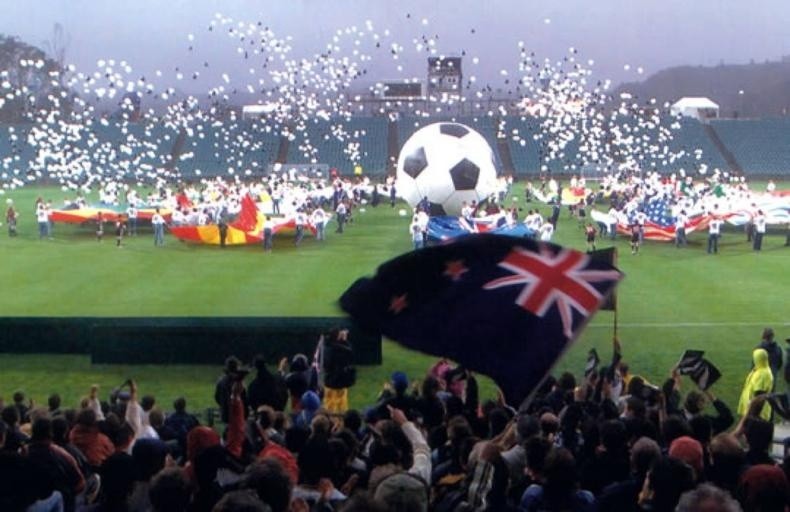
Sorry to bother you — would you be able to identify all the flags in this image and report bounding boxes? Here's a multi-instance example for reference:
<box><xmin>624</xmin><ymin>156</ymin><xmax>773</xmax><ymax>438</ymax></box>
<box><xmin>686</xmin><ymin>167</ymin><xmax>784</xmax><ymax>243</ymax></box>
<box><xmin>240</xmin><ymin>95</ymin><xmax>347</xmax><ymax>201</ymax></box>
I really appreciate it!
<box><xmin>337</xmin><ymin>217</ymin><xmax>624</xmax><ymax>414</ymax></box>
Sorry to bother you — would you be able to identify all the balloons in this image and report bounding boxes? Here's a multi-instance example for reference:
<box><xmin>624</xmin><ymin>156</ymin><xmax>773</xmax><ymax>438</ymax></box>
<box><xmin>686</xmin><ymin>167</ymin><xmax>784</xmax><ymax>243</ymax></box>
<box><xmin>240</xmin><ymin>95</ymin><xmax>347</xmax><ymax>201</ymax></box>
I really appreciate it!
<box><xmin>0</xmin><ymin>9</ymin><xmax>748</xmax><ymax>206</ymax></box>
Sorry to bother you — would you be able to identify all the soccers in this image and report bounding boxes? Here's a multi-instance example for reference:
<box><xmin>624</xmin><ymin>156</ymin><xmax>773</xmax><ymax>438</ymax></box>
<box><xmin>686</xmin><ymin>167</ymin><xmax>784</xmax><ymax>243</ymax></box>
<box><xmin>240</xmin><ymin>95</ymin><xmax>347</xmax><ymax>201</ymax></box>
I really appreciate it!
<box><xmin>397</xmin><ymin>122</ymin><xmax>498</xmax><ymax>219</ymax></box>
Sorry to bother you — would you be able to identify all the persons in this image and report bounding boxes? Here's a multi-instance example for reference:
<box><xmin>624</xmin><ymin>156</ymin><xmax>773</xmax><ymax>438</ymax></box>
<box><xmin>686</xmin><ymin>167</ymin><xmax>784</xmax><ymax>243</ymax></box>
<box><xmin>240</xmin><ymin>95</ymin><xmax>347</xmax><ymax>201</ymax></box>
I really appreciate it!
<box><xmin>2</xmin><ymin>162</ymin><xmax>396</xmax><ymax>254</ymax></box>
<box><xmin>409</xmin><ymin>161</ymin><xmax>790</xmax><ymax>255</ymax></box>
<box><xmin>1</xmin><ymin>324</ymin><xmax>790</xmax><ymax>511</ymax></box>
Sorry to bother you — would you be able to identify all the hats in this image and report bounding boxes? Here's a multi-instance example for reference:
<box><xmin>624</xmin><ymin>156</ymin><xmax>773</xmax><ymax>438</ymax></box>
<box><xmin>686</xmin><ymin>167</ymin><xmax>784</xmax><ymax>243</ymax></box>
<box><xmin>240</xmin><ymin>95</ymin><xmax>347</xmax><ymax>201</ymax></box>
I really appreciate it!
<box><xmin>133</xmin><ymin>437</ymin><xmax>168</xmax><ymax>478</ymax></box>
<box><xmin>373</xmin><ymin>473</ymin><xmax>429</xmax><ymax>512</ymax></box>
<box><xmin>300</xmin><ymin>391</ymin><xmax>321</xmax><ymax>412</ymax></box>
<box><xmin>392</xmin><ymin>372</ymin><xmax>409</xmax><ymax>389</ymax></box>
<box><xmin>521</xmin><ymin>484</ymin><xmax>598</xmax><ymax>511</ymax></box>
<box><xmin>668</xmin><ymin>436</ymin><xmax>704</xmax><ymax>469</ymax></box>
<box><xmin>737</xmin><ymin>465</ymin><xmax>789</xmax><ymax>510</ymax></box>
